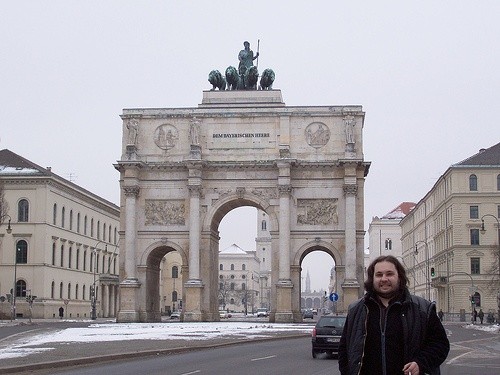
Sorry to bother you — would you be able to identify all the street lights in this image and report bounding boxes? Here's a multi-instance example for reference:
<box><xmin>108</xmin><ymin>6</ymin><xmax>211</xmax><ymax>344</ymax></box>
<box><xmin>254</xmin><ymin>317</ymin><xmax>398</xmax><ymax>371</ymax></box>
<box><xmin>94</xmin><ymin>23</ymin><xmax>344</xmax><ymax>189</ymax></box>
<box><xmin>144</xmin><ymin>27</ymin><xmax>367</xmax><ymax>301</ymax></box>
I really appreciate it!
<box><xmin>13</xmin><ymin>239</ymin><xmax>21</xmax><ymax>320</ymax></box>
<box><xmin>92</xmin><ymin>241</ymin><xmax>108</xmax><ymax>319</ymax></box>
<box><xmin>415</xmin><ymin>241</ymin><xmax>431</xmax><ymax>304</ymax></box>
<box><xmin>480</xmin><ymin>214</ymin><xmax>500</xmax><ymax>291</ymax></box>
<box><xmin>245</xmin><ymin>270</ymin><xmax>254</xmax><ymax>315</ymax></box>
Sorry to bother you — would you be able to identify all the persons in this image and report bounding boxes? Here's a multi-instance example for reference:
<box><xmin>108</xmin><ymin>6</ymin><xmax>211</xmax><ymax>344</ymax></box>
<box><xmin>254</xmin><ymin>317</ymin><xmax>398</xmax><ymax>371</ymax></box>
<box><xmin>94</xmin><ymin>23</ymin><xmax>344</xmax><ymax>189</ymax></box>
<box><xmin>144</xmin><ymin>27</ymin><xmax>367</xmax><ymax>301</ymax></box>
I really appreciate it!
<box><xmin>56</xmin><ymin>305</ymin><xmax>67</xmax><ymax>319</ymax></box>
<box><xmin>473</xmin><ymin>308</ymin><xmax>478</xmax><ymax>321</ymax></box>
<box><xmin>338</xmin><ymin>254</ymin><xmax>451</xmax><ymax>375</ymax></box>
<box><xmin>236</xmin><ymin>41</ymin><xmax>260</xmax><ymax>75</ymax></box>
<box><xmin>479</xmin><ymin>309</ymin><xmax>484</xmax><ymax>325</ymax></box>
<box><xmin>438</xmin><ymin>307</ymin><xmax>445</xmax><ymax>321</ymax></box>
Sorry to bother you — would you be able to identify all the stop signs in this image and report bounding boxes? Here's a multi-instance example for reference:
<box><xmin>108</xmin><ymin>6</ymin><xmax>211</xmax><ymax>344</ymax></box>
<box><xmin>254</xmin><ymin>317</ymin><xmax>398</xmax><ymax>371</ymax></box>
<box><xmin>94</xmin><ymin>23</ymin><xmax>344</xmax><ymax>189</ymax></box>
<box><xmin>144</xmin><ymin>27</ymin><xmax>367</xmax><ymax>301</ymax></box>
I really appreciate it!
<box><xmin>64</xmin><ymin>299</ymin><xmax>69</xmax><ymax>304</ymax></box>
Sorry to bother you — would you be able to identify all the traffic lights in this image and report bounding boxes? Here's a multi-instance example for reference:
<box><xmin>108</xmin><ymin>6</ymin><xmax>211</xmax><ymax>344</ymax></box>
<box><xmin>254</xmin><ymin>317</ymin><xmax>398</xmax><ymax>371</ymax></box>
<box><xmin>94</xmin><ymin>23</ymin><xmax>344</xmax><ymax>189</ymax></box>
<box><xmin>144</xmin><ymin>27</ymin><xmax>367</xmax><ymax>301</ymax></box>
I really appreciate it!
<box><xmin>431</xmin><ymin>268</ymin><xmax>435</xmax><ymax>276</ymax></box>
<box><xmin>471</xmin><ymin>294</ymin><xmax>475</xmax><ymax>304</ymax></box>
<box><xmin>32</xmin><ymin>296</ymin><xmax>37</xmax><ymax>302</ymax></box>
<box><xmin>5</xmin><ymin>293</ymin><xmax>11</xmax><ymax>302</ymax></box>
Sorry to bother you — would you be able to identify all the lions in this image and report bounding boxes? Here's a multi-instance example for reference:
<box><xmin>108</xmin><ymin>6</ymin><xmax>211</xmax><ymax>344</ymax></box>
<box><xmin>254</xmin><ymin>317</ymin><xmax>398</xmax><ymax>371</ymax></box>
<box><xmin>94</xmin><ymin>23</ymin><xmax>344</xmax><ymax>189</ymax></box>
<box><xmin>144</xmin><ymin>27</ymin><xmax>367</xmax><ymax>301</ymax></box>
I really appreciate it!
<box><xmin>208</xmin><ymin>65</ymin><xmax>275</xmax><ymax>91</ymax></box>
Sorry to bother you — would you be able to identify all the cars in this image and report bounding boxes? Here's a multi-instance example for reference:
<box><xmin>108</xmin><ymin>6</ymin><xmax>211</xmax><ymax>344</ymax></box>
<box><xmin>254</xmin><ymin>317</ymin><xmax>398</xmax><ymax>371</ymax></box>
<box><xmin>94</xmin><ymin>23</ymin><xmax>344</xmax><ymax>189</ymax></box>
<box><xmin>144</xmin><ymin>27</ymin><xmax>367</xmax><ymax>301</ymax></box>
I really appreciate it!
<box><xmin>220</xmin><ymin>307</ymin><xmax>334</xmax><ymax>319</ymax></box>
<box><xmin>170</xmin><ymin>309</ymin><xmax>182</xmax><ymax>319</ymax></box>
<box><xmin>311</xmin><ymin>315</ymin><xmax>347</xmax><ymax>359</ymax></box>
